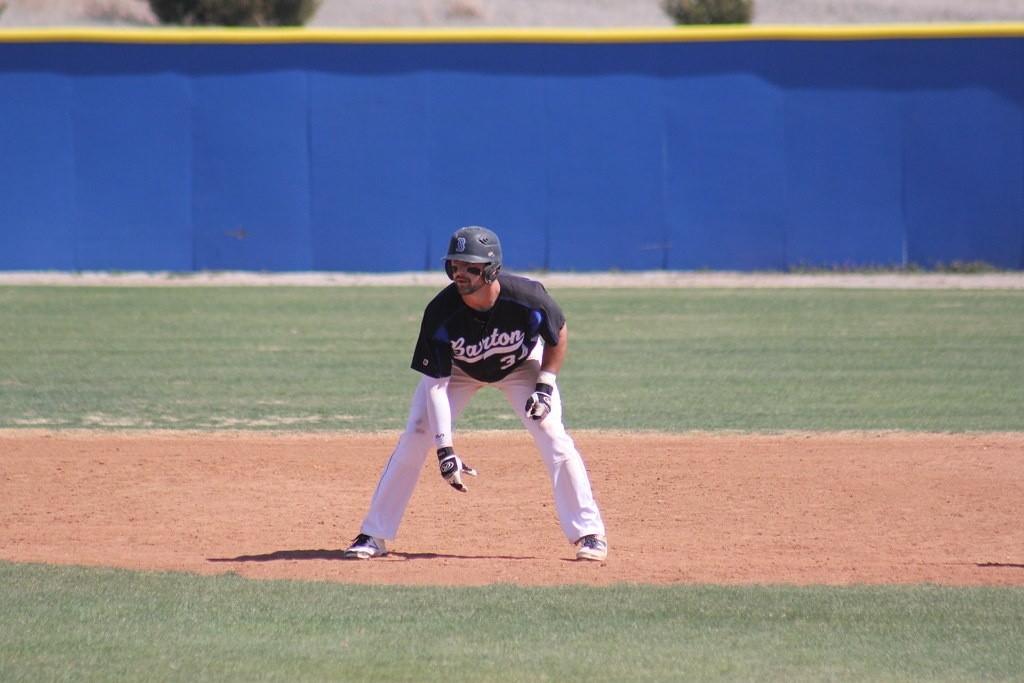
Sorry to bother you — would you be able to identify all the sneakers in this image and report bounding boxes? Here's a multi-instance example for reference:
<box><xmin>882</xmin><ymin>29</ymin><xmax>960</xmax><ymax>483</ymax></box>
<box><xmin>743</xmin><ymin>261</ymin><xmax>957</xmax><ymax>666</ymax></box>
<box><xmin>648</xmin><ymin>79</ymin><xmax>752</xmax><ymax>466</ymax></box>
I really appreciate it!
<box><xmin>577</xmin><ymin>534</ymin><xmax>608</xmax><ymax>560</ymax></box>
<box><xmin>345</xmin><ymin>533</ymin><xmax>386</xmax><ymax>559</ymax></box>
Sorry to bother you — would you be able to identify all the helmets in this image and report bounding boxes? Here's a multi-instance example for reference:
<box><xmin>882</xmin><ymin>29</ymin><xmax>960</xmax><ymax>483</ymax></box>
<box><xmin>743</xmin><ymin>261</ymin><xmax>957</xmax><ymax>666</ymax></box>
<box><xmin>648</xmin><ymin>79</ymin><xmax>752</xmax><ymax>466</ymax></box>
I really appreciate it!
<box><xmin>440</xmin><ymin>226</ymin><xmax>503</xmax><ymax>284</ymax></box>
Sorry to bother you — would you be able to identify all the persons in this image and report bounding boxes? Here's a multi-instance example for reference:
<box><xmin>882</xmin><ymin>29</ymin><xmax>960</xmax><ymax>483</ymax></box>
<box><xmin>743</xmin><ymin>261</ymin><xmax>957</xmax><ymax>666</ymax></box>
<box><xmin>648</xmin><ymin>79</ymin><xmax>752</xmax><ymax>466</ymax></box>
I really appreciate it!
<box><xmin>343</xmin><ymin>225</ymin><xmax>608</xmax><ymax>561</ymax></box>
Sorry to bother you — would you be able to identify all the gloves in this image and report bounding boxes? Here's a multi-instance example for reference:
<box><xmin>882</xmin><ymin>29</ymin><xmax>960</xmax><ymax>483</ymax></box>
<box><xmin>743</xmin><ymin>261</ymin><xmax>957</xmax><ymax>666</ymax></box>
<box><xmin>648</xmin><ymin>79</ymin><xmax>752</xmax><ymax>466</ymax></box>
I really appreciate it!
<box><xmin>524</xmin><ymin>370</ymin><xmax>558</xmax><ymax>422</ymax></box>
<box><xmin>437</xmin><ymin>446</ymin><xmax>476</xmax><ymax>493</ymax></box>
